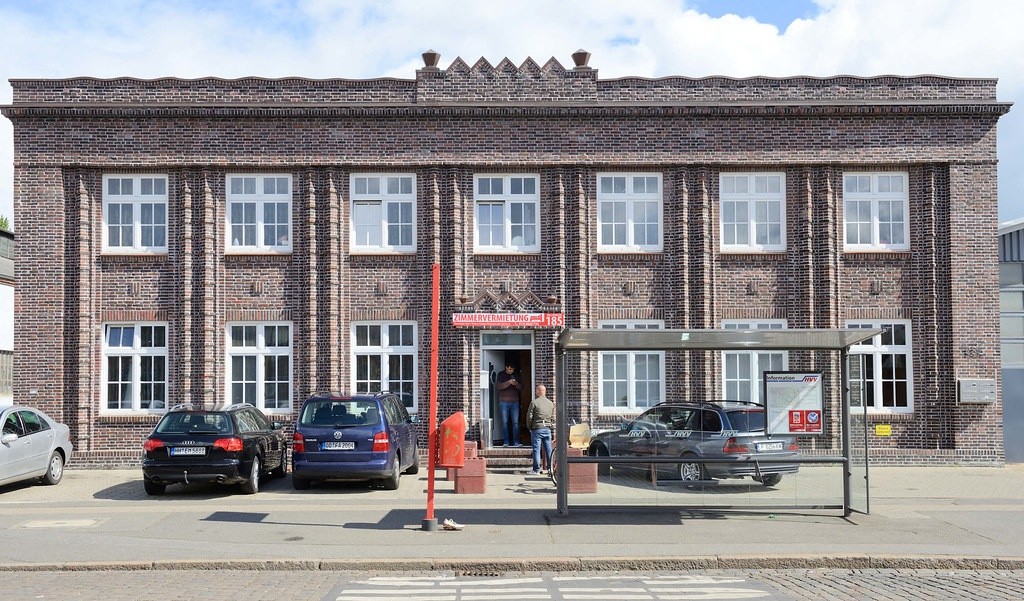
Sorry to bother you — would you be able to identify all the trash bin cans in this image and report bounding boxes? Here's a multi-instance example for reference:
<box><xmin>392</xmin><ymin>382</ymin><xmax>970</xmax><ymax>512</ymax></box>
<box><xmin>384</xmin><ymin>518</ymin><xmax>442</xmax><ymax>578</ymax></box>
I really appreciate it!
<box><xmin>479</xmin><ymin>417</ymin><xmax>493</xmax><ymax>449</ymax></box>
<box><xmin>439</xmin><ymin>410</ymin><xmax>465</xmax><ymax>469</ymax></box>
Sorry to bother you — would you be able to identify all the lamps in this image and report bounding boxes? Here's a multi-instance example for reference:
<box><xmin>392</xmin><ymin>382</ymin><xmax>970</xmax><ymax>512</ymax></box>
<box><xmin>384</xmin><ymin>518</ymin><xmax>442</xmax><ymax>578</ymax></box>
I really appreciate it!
<box><xmin>749</xmin><ymin>280</ymin><xmax>759</xmax><ymax>293</ymax></box>
<box><xmin>253</xmin><ymin>281</ymin><xmax>263</xmax><ymax>295</ymax></box>
<box><xmin>378</xmin><ymin>281</ymin><xmax>387</xmax><ymax>294</ymax></box>
<box><xmin>626</xmin><ymin>281</ymin><xmax>635</xmax><ymax>295</ymax></box>
<box><xmin>872</xmin><ymin>281</ymin><xmax>883</xmax><ymax>294</ymax></box>
<box><xmin>129</xmin><ymin>282</ymin><xmax>139</xmax><ymax>297</ymax></box>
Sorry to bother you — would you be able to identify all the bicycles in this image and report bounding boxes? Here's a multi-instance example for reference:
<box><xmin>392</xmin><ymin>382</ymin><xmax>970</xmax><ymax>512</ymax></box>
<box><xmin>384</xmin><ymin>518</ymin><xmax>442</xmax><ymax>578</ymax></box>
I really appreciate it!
<box><xmin>550</xmin><ymin>418</ymin><xmax>577</xmax><ymax>485</ymax></box>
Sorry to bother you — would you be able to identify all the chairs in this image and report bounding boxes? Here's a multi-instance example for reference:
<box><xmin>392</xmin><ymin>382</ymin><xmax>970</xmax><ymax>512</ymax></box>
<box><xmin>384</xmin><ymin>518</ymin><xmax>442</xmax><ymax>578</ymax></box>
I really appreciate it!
<box><xmin>365</xmin><ymin>409</ymin><xmax>376</xmax><ymax>422</ymax></box>
<box><xmin>190</xmin><ymin>416</ymin><xmax>204</xmax><ymax>425</ymax></box>
<box><xmin>331</xmin><ymin>405</ymin><xmax>347</xmax><ymax>421</ymax></box>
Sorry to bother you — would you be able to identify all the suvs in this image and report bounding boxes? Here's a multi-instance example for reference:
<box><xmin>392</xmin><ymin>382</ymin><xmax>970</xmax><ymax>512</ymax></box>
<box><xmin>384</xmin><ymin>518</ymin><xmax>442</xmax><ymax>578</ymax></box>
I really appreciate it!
<box><xmin>141</xmin><ymin>403</ymin><xmax>288</xmax><ymax>494</ymax></box>
<box><xmin>587</xmin><ymin>400</ymin><xmax>803</xmax><ymax>488</ymax></box>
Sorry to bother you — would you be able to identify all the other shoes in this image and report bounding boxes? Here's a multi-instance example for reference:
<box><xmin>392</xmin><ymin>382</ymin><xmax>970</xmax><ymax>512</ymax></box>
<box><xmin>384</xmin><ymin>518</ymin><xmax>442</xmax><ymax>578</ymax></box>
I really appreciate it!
<box><xmin>527</xmin><ymin>470</ymin><xmax>540</xmax><ymax>474</ymax></box>
<box><xmin>548</xmin><ymin>468</ymin><xmax>551</xmax><ymax>474</ymax></box>
<box><xmin>513</xmin><ymin>442</ymin><xmax>523</xmax><ymax>446</ymax></box>
<box><xmin>503</xmin><ymin>443</ymin><xmax>508</xmax><ymax>447</ymax></box>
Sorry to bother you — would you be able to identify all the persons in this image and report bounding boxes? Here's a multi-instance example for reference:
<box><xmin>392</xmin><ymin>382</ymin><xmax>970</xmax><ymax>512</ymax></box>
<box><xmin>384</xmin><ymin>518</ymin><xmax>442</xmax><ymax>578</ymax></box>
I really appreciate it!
<box><xmin>496</xmin><ymin>361</ymin><xmax>524</xmax><ymax>447</ymax></box>
<box><xmin>526</xmin><ymin>385</ymin><xmax>554</xmax><ymax>475</ymax></box>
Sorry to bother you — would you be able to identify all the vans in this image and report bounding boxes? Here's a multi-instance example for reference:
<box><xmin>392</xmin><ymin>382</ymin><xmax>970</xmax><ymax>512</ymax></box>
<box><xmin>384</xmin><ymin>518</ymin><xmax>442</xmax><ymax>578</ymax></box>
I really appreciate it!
<box><xmin>292</xmin><ymin>391</ymin><xmax>419</xmax><ymax>489</ymax></box>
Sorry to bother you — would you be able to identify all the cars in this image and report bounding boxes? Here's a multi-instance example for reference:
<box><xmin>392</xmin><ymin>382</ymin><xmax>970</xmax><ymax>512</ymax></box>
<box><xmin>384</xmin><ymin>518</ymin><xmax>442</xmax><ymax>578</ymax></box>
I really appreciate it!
<box><xmin>0</xmin><ymin>406</ymin><xmax>73</xmax><ymax>485</ymax></box>
